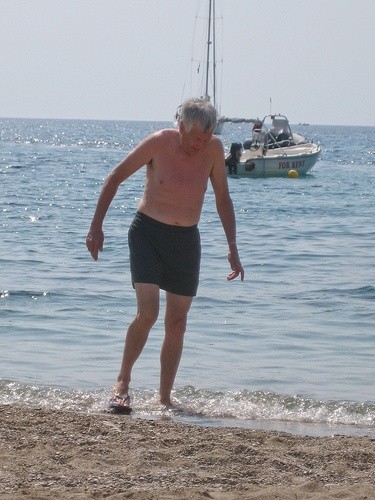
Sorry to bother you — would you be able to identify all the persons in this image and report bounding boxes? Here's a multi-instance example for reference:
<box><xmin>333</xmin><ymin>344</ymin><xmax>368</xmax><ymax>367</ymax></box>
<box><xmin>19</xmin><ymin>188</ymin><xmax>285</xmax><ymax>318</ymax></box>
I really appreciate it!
<box><xmin>252</xmin><ymin>118</ymin><xmax>263</xmax><ymax>134</ymax></box>
<box><xmin>85</xmin><ymin>98</ymin><xmax>246</xmax><ymax>414</ymax></box>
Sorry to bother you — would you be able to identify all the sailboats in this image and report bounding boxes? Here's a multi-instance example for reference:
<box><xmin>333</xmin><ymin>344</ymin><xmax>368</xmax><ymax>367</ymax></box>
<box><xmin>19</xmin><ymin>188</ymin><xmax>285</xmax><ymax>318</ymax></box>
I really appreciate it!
<box><xmin>170</xmin><ymin>0</ymin><xmax>227</xmax><ymax>144</ymax></box>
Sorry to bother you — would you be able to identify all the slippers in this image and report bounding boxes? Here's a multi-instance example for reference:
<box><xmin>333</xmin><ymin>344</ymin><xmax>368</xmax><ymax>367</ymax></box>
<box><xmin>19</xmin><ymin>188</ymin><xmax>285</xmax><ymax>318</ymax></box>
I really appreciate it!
<box><xmin>109</xmin><ymin>393</ymin><xmax>133</xmax><ymax>412</ymax></box>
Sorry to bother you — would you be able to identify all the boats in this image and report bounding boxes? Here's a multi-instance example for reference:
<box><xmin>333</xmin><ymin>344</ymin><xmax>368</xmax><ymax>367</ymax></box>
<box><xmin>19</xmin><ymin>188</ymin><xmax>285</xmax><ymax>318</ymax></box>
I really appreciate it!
<box><xmin>224</xmin><ymin>110</ymin><xmax>323</xmax><ymax>179</ymax></box>
